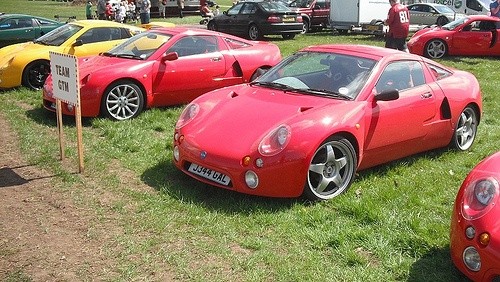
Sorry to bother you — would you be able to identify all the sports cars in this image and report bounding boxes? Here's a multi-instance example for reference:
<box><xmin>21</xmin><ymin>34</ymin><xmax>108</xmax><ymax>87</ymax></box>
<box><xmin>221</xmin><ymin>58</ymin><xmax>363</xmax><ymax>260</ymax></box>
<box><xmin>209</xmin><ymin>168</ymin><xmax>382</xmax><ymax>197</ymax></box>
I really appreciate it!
<box><xmin>0</xmin><ymin>19</ymin><xmax>176</xmax><ymax>92</ymax></box>
<box><xmin>173</xmin><ymin>43</ymin><xmax>482</xmax><ymax>205</ymax></box>
<box><xmin>42</xmin><ymin>25</ymin><xmax>282</xmax><ymax>122</ymax></box>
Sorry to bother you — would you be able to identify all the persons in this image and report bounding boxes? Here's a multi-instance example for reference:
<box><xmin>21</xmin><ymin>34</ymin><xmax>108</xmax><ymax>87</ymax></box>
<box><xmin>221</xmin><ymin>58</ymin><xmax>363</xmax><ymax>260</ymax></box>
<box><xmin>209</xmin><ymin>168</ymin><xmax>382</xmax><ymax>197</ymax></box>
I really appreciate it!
<box><xmin>385</xmin><ymin>0</ymin><xmax>411</xmax><ymax>51</ymax></box>
<box><xmin>490</xmin><ymin>0</ymin><xmax>500</xmax><ymax>17</ymax></box>
<box><xmin>157</xmin><ymin>0</ymin><xmax>167</xmax><ymax>19</ymax></box>
<box><xmin>200</xmin><ymin>0</ymin><xmax>220</xmax><ymax>21</ymax></box>
<box><xmin>177</xmin><ymin>0</ymin><xmax>185</xmax><ymax>18</ymax></box>
<box><xmin>138</xmin><ymin>0</ymin><xmax>151</xmax><ymax>25</ymax></box>
<box><xmin>85</xmin><ymin>0</ymin><xmax>136</xmax><ymax>23</ymax></box>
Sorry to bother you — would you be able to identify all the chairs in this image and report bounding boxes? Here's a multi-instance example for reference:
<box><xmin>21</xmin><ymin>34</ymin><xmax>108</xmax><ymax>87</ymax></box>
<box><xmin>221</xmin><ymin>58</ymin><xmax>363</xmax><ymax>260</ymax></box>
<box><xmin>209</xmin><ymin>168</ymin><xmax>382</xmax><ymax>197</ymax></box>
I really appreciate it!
<box><xmin>311</xmin><ymin>56</ymin><xmax>359</xmax><ymax>93</ymax></box>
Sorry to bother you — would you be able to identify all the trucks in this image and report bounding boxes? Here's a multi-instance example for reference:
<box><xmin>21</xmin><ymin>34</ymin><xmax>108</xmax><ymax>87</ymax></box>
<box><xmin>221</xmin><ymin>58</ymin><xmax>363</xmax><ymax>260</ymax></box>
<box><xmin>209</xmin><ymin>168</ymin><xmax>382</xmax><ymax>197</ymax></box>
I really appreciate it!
<box><xmin>330</xmin><ymin>0</ymin><xmax>400</xmax><ymax>37</ymax></box>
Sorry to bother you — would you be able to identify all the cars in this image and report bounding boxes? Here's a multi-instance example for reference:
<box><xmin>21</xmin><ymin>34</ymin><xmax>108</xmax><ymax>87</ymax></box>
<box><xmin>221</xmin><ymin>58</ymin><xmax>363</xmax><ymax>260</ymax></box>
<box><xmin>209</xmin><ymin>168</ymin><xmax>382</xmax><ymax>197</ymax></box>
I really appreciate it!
<box><xmin>406</xmin><ymin>14</ymin><xmax>500</xmax><ymax>59</ymax></box>
<box><xmin>0</xmin><ymin>12</ymin><xmax>67</xmax><ymax>48</ymax></box>
<box><xmin>288</xmin><ymin>0</ymin><xmax>330</xmax><ymax>35</ymax></box>
<box><xmin>206</xmin><ymin>0</ymin><xmax>304</xmax><ymax>41</ymax></box>
<box><xmin>405</xmin><ymin>2</ymin><xmax>468</xmax><ymax>27</ymax></box>
